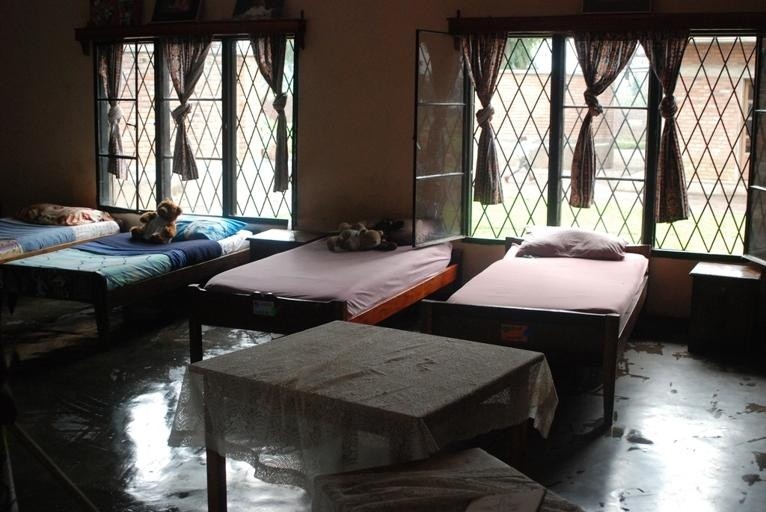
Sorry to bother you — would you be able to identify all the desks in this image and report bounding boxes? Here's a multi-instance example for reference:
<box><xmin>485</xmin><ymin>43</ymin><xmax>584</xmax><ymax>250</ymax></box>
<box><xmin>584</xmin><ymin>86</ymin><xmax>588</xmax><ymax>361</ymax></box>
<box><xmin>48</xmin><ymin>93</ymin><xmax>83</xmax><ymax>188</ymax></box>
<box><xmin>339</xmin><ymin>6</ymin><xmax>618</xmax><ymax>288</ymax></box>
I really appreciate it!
<box><xmin>188</xmin><ymin>320</ymin><xmax>559</xmax><ymax>512</ymax></box>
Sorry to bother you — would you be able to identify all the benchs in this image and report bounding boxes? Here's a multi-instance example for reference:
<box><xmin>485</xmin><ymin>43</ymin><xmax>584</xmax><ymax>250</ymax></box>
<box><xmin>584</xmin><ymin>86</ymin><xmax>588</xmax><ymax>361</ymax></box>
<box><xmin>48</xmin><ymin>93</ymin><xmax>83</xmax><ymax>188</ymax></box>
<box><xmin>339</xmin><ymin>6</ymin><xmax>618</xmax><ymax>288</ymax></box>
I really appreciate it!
<box><xmin>314</xmin><ymin>448</ymin><xmax>585</xmax><ymax>512</ymax></box>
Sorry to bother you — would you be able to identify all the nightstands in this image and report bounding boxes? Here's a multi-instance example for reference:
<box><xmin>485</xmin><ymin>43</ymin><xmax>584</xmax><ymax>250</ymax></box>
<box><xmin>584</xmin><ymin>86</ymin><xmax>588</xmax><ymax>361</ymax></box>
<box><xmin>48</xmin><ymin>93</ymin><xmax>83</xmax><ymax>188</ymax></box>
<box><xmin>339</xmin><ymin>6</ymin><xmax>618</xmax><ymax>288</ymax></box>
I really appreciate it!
<box><xmin>688</xmin><ymin>262</ymin><xmax>762</xmax><ymax>352</ymax></box>
<box><xmin>247</xmin><ymin>228</ymin><xmax>325</xmax><ymax>261</ymax></box>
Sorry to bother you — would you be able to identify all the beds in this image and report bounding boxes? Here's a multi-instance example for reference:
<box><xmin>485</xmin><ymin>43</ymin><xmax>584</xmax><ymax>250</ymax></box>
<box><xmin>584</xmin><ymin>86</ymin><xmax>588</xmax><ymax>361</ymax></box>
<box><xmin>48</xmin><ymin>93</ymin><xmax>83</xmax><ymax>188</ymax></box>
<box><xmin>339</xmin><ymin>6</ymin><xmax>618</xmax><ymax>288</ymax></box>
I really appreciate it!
<box><xmin>186</xmin><ymin>232</ymin><xmax>459</xmax><ymax>365</ymax></box>
<box><xmin>420</xmin><ymin>238</ymin><xmax>653</xmax><ymax>436</ymax></box>
<box><xmin>0</xmin><ymin>219</ymin><xmax>124</xmax><ymax>314</ymax></box>
<box><xmin>0</xmin><ymin>229</ymin><xmax>269</xmax><ymax>354</ymax></box>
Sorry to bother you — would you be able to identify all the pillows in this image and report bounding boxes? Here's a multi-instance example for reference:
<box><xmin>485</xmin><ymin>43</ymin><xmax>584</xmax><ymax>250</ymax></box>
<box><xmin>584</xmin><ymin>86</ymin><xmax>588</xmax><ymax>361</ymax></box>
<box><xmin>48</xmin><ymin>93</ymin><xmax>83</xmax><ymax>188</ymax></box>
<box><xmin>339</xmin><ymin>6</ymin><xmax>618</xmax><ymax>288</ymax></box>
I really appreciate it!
<box><xmin>16</xmin><ymin>203</ymin><xmax>80</xmax><ymax>226</ymax></box>
<box><xmin>517</xmin><ymin>240</ymin><xmax>626</xmax><ymax>260</ymax></box>
<box><xmin>362</xmin><ymin>218</ymin><xmax>442</xmax><ymax>244</ymax></box>
<box><xmin>172</xmin><ymin>218</ymin><xmax>247</xmax><ymax>240</ymax></box>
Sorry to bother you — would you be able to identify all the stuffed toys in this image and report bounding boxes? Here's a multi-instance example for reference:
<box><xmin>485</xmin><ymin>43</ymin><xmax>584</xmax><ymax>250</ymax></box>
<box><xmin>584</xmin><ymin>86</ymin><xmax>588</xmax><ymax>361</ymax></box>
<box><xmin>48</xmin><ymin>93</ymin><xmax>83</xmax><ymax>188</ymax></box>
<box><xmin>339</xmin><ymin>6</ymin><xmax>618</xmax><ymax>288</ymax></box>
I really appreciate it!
<box><xmin>371</xmin><ymin>218</ymin><xmax>404</xmax><ymax>250</ymax></box>
<box><xmin>327</xmin><ymin>221</ymin><xmax>383</xmax><ymax>250</ymax></box>
<box><xmin>130</xmin><ymin>198</ymin><xmax>182</xmax><ymax>244</ymax></box>
